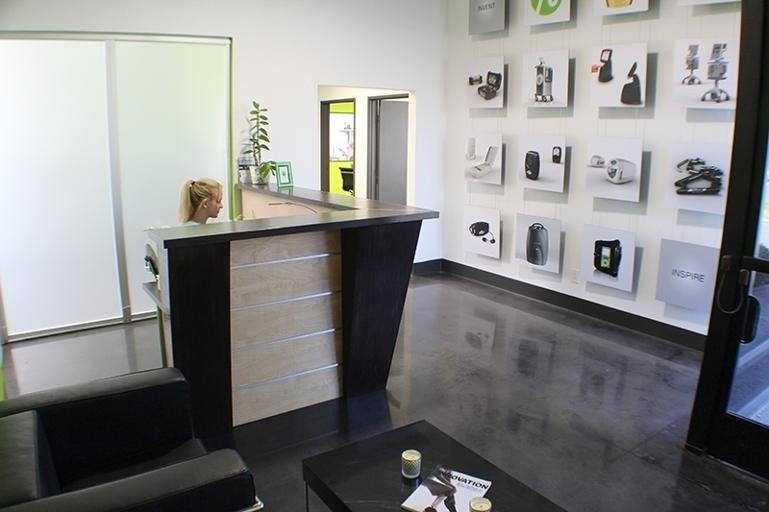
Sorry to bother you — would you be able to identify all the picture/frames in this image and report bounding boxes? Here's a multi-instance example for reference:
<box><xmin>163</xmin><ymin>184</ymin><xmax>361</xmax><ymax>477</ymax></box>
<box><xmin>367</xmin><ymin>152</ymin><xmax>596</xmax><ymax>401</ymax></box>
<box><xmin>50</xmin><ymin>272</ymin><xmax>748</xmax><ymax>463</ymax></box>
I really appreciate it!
<box><xmin>276</xmin><ymin>163</ymin><xmax>293</xmax><ymax>188</ymax></box>
<box><xmin>328</xmin><ymin>110</ymin><xmax>355</xmax><ymax>161</ymax></box>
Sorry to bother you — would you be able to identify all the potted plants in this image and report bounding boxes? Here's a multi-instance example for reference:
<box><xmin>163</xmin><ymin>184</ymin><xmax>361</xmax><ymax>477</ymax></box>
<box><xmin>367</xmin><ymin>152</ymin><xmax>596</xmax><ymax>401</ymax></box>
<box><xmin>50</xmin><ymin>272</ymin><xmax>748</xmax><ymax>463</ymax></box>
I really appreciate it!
<box><xmin>243</xmin><ymin>100</ymin><xmax>276</xmax><ymax>186</ymax></box>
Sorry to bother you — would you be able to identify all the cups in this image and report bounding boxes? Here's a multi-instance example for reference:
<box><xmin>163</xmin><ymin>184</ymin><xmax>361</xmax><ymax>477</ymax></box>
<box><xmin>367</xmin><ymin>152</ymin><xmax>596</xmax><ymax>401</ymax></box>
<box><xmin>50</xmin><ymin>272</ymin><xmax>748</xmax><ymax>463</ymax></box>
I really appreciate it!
<box><xmin>401</xmin><ymin>450</ymin><xmax>422</xmax><ymax>478</ymax></box>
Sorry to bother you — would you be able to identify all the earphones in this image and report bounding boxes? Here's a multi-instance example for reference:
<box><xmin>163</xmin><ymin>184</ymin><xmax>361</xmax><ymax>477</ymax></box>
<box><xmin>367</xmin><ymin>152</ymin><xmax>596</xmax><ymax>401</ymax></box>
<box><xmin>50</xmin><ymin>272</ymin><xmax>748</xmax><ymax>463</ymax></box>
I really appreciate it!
<box><xmin>482</xmin><ymin>237</ymin><xmax>487</xmax><ymax>241</ymax></box>
<box><xmin>490</xmin><ymin>239</ymin><xmax>495</xmax><ymax>243</ymax></box>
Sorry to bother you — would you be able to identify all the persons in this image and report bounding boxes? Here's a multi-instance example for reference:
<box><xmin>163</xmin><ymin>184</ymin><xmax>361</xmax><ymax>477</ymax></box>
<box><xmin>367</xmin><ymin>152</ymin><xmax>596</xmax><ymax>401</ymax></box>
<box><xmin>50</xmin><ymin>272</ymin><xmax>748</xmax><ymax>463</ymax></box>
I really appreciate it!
<box><xmin>422</xmin><ymin>465</ymin><xmax>457</xmax><ymax>512</ymax></box>
<box><xmin>181</xmin><ymin>179</ymin><xmax>223</xmax><ymax>227</ymax></box>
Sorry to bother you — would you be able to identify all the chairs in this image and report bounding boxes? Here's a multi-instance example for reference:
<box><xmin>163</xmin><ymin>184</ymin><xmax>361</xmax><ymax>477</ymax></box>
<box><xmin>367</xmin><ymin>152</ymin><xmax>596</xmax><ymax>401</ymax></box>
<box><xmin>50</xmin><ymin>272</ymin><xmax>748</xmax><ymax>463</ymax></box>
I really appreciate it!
<box><xmin>340</xmin><ymin>167</ymin><xmax>354</xmax><ymax>193</ymax></box>
<box><xmin>0</xmin><ymin>369</ymin><xmax>265</xmax><ymax>512</ymax></box>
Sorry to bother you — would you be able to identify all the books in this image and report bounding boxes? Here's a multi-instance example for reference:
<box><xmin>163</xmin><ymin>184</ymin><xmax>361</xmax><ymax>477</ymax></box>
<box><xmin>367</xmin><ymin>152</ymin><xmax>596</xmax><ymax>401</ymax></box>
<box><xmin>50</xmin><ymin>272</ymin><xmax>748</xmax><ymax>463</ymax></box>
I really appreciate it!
<box><xmin>401</xmin><ymin>463</ymin><xmax>492</xmax><ymax>512</ymax></box>
